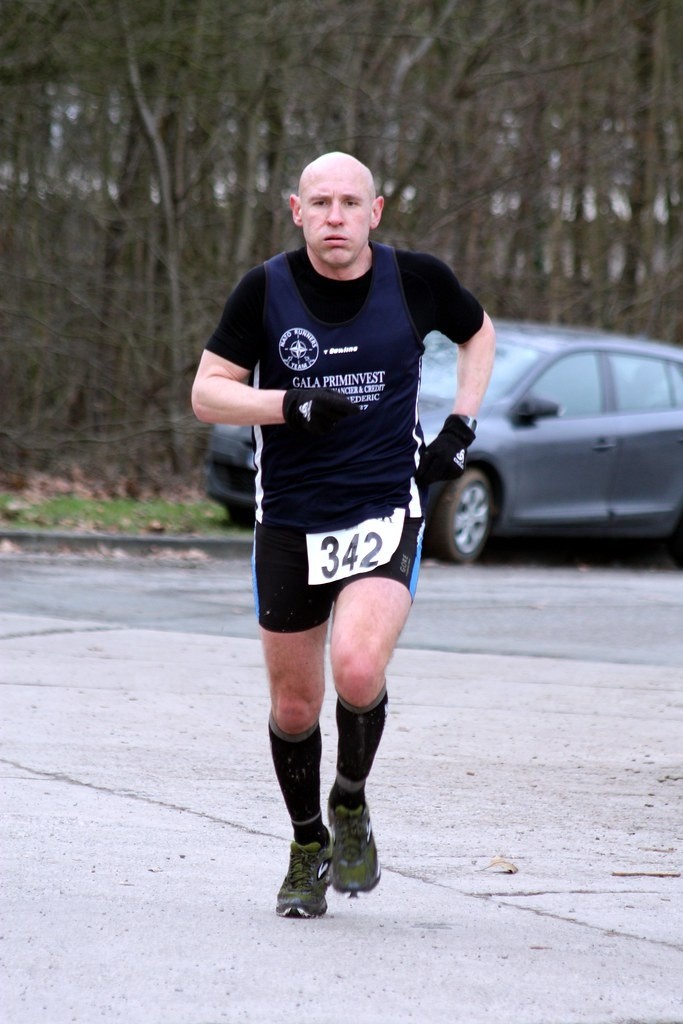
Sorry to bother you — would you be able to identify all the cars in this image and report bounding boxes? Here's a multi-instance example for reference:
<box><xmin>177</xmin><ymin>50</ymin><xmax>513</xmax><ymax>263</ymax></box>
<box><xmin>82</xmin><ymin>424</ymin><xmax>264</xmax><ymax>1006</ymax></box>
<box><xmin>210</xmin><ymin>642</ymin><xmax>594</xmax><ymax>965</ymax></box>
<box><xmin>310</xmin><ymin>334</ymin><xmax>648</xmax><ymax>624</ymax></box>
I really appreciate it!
<box><xmin>203</xmin><ymin>317</ymin><xmax>682</xmax><ymax>564</ymax></box>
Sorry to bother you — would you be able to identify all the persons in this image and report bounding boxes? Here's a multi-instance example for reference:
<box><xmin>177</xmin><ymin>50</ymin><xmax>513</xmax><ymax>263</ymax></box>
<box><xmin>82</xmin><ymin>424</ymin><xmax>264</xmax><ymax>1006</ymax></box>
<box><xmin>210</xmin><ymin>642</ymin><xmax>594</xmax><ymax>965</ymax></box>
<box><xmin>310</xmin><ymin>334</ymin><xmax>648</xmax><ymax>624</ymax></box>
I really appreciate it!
<box><xmin>191</xmin><ymin>152</ymin><xmax>497</xmax><ymax>918</ymax></box>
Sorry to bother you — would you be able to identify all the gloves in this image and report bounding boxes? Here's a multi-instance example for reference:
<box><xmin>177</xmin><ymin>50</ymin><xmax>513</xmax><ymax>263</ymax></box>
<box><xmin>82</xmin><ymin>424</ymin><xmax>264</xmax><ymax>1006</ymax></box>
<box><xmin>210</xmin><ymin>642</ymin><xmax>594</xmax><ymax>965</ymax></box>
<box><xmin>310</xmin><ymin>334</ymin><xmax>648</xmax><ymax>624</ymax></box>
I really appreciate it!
<box><xmin>282</xmin><ymin>387</ymin><xmax>360</xmax><ymax>440</ymax></box>
<box><xmin>414</xmin><ymin>414</ymin><xmax>476</xmax><ymax>491</ymax></box>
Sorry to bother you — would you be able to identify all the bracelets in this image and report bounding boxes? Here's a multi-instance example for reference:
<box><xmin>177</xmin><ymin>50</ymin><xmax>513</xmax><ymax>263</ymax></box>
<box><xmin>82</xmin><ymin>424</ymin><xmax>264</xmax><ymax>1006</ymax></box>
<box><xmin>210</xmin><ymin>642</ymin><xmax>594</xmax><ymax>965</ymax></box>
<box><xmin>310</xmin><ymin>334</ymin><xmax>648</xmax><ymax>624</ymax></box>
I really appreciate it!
<box><xmin>460</xmin><ymin>415</ymin><xmax>477</xmax><ymax>432</ymax></box>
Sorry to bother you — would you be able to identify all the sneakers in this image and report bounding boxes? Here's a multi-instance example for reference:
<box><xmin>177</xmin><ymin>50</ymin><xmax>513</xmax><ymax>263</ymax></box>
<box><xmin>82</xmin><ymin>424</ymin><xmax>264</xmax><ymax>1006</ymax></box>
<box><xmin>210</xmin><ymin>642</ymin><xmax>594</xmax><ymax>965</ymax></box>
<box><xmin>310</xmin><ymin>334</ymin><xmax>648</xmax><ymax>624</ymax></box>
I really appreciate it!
<box><xmin>275</xmin><ymin>825</ymin><xmax>334</xmax><ymax>918</ymax></box>
<box><xmin>327</xmin><ymin>787</ymin><xmax>382</xmax><ymax>898</ymax></box>
